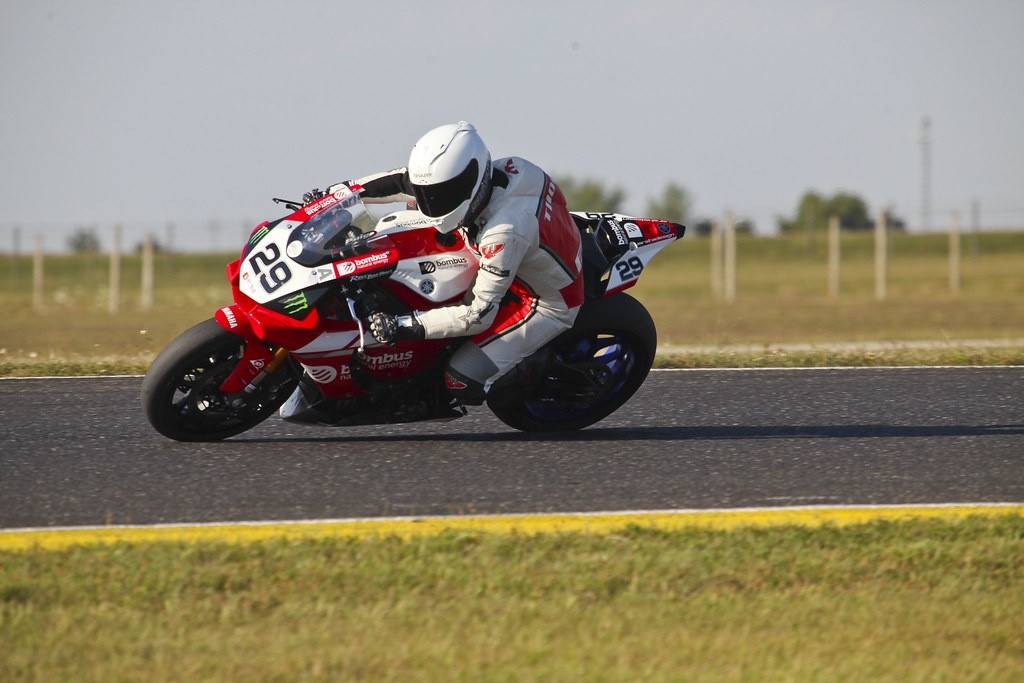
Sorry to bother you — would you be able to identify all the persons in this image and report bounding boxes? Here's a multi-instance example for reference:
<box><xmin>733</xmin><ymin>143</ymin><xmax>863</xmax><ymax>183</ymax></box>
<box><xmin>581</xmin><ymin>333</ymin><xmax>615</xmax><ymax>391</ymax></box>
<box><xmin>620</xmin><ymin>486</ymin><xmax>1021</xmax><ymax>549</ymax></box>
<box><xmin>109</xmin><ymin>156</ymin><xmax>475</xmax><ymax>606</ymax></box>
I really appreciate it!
<box><xmin>300</xmin><ymin>121</ymin><xmax>584</xmax><ymax>406</ymax></box>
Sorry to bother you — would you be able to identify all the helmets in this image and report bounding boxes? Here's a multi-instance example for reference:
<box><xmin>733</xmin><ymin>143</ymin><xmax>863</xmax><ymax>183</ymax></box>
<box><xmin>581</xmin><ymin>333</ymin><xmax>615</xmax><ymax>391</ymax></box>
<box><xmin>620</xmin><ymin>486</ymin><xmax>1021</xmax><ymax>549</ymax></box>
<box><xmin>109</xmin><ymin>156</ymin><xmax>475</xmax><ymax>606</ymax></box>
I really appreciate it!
<box><xmin>408</xmin><ymin>120</ymin><xmax>494</xmax><ymax>234</ymax></box>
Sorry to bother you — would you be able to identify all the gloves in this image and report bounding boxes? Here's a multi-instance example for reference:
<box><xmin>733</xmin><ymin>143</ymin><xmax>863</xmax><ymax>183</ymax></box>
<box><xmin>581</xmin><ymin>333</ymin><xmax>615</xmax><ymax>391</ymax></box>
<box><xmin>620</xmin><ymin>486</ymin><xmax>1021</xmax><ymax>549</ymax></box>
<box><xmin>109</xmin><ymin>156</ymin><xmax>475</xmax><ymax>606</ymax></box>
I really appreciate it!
<box><xmin>302</xmin><ymin>180</ymin><xmax>356</xmax><ymax>207</ymax></box>
<box><xmin>367</xmin><ymin>309</ymin><xmax>428</xmax><ymax>344</ymax></box>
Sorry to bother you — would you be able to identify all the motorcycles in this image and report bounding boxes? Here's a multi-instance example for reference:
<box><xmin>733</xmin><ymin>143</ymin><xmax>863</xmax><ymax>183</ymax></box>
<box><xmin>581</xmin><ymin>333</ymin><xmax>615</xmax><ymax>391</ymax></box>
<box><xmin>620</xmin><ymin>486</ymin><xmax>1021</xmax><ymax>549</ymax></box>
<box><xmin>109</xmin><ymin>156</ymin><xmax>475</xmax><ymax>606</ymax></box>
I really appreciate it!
<box><xmin>138</xmin><ymin>180</ymin><xmax>688</xmax><ymax>443</ymax></box>
